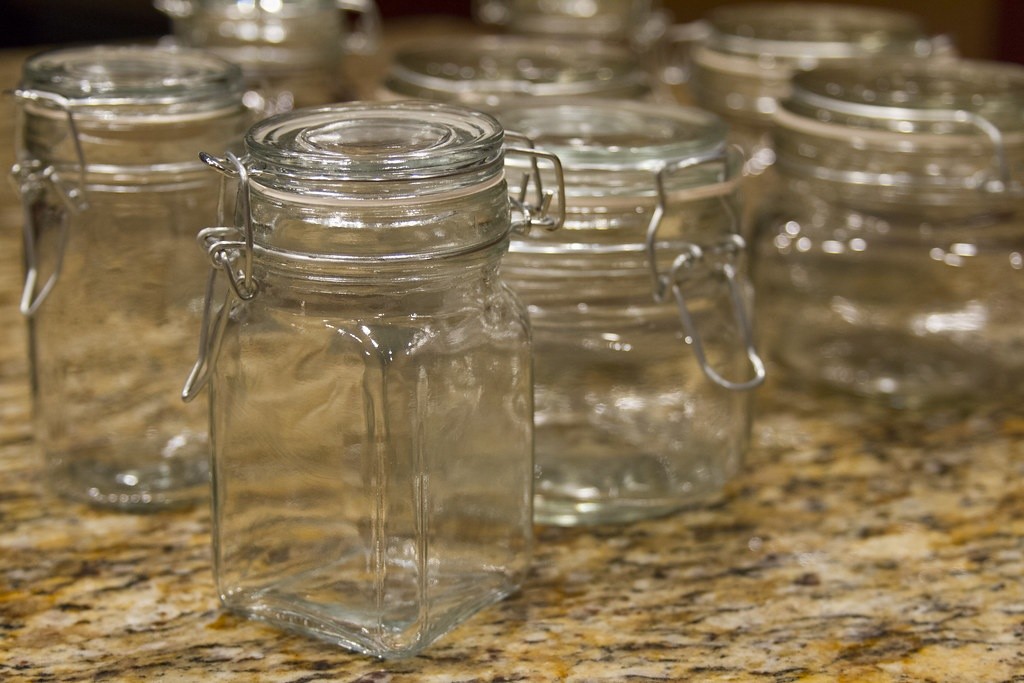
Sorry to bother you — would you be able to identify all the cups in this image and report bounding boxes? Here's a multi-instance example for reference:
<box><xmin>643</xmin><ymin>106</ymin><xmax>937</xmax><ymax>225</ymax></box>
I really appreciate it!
<box><xmin>19</xmin><ymin>1</ymin><xmax>1024</xmax><ymax>660</ymax></box>
